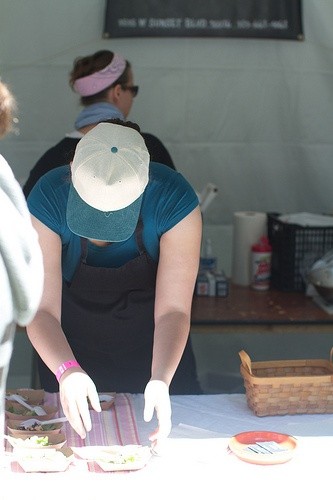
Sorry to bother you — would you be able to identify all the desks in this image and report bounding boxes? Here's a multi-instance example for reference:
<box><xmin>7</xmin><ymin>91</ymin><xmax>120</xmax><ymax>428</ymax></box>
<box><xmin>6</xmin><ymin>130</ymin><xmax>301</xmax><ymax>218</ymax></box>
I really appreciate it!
<box><xmin>4</xmin><ymin>389</ymin><xmax>333</xmax><ymax>500</ymax></box>
<box><xmin>190</xmin><ymin>277</ymin><xmax>333</xmax><ymax>394</ymax></box>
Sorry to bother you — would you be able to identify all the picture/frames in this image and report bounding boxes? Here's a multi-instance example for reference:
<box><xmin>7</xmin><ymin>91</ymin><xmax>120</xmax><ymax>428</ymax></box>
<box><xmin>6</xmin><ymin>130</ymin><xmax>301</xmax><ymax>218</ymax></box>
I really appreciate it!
<box><xmin>103</xmin><ymin>0</ymin><xmax>306</xmax><ymax>42</ymax></box>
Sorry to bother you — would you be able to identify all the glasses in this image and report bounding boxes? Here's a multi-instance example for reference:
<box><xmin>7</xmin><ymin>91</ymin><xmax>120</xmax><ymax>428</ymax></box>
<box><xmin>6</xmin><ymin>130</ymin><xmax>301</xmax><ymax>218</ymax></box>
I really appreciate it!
<box><xmin>122</xmin><ymin>85</ymin><xmax>138</xmax><ymax>98</ymax></box>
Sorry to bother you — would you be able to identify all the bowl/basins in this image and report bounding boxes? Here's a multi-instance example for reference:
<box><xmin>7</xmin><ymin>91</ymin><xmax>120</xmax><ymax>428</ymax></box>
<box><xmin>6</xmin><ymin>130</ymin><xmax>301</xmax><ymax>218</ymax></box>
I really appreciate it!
<box><xmin>228</xmin><ymin>431</ymin><xmax>298</xmax><ymax>464</ymax></box>
<box><xmin>5</xmin><ymin>389</ymin><xmax>74</xmax><ymax>472</ymax></box>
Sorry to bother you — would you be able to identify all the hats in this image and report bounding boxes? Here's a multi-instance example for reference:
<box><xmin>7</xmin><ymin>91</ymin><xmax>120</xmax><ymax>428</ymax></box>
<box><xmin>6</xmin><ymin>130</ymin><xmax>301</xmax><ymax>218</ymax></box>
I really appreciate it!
<box><xmin>66</xmin><ymin>122</ymin><xmax>150</xmax><ymax>242</ymax></box>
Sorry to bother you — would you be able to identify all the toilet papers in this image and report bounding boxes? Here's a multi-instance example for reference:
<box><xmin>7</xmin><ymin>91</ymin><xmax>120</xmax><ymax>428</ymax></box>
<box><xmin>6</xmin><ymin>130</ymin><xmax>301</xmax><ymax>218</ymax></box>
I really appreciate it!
<box><xmin>230</xmin><ymin>210</ymin><xmax>269</xmax><ymax>288</ymax></box>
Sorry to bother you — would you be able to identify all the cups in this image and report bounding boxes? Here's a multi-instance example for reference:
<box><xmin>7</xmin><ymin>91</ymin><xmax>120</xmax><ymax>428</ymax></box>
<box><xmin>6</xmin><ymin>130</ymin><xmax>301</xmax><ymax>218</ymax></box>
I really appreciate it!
<box><xmin>251</xmin><ymin>237</ymin><xmax>272</xmax><ymax>289</ymax></box>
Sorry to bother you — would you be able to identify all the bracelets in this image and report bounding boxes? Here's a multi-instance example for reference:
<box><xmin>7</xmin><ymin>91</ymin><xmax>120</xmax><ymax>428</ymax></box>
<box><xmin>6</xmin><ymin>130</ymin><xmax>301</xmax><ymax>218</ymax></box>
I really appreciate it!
<box><xmin>56</xmin><ymin>361</ymin><xmax>80</xmax><ymax>381</ymax></box>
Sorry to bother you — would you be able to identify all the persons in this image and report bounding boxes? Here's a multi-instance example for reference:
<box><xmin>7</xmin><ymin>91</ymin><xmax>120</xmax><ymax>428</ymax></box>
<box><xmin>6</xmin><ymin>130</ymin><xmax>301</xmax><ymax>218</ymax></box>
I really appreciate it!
<box><xmin>0</xmin><ymin>82</ymin><xmax>44</xmax><ymax>388</ymax></box>
<box><xmin>27</xmin><ymin>122</ymin><xmax>202</xmax><ymax>455</ymax></box>
<box><xmin>24</xmin><ymin>50</ymin><xmax>179</xmax><ymax>201</ymax></box>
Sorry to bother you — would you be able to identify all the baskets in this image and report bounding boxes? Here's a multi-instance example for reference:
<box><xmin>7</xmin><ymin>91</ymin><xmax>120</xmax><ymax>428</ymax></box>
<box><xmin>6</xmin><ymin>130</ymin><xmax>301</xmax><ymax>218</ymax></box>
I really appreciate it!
<box><xmin>238</xmin><ymin>350</ymin><xmax>333</xmax><ymax>416</ymax></box>
<box><xmin>266</xmin><ymin>210</ymin><xmax>333</xmax><ymax>305</ymax></box>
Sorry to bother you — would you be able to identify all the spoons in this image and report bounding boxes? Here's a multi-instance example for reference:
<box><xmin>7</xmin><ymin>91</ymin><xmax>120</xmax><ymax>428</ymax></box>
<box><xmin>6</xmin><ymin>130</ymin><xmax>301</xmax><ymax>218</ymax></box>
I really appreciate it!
<box><xmin>15</xmin><ymin>398</ymin><xmax>46</xmax><ymax>415</ymax></box>
<box><xmin>21</xmin><ymin>418</ymin><xmax>68</xmax><ymax>426</ymax></box>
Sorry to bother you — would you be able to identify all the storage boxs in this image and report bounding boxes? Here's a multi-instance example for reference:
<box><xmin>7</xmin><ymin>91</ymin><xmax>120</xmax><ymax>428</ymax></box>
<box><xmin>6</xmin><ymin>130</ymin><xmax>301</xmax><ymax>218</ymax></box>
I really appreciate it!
<box><xmin>195</xmin><ymin>268</ymin><xmax>228</xmax><ymax>296</ymax></box>
<box><xmin>267</xmin><ymin>211</ymin><xmax>333</xmax><ymax>293</ymax></box>
